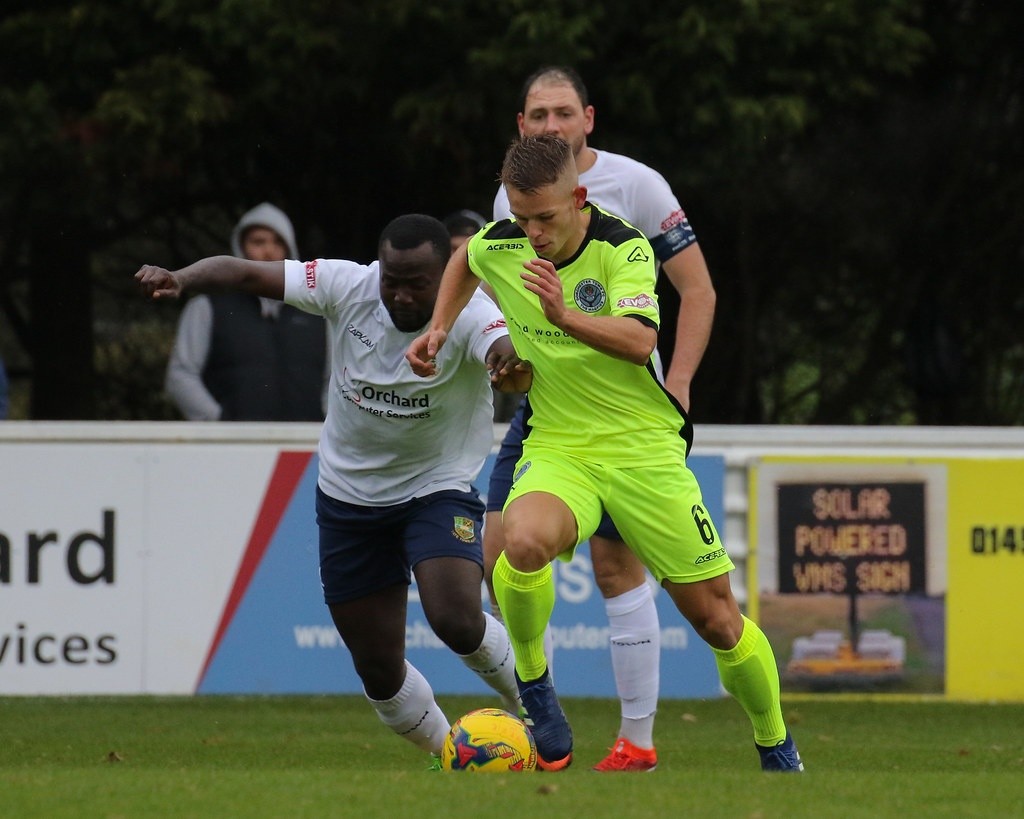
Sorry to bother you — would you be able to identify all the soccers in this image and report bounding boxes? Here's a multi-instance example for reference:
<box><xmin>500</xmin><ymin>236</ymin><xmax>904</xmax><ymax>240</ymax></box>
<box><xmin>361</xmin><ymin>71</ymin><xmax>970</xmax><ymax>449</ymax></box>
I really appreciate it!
<box><xmin>441</xmin><ymin>707</ymin><xmax>538</xmax><ymax>772</ymax></box>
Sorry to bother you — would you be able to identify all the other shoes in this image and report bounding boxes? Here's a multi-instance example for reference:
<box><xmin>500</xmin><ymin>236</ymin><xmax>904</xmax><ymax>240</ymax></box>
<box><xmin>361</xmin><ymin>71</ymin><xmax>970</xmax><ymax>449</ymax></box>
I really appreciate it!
<box><xmin>431</xmin><ymin>755</ymin><xmax>445</xmax><ymax>772</ymax></box>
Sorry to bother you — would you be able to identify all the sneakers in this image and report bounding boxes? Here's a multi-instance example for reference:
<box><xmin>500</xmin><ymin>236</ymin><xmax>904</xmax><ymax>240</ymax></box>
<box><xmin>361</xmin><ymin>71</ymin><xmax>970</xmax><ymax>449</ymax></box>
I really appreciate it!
<box><xmin>515</xmin><ymin>665</ymin><xmax>573</xmax><ymax>771</ymax></box>
<box><xmin>592</xmin><ymin>738</ymin><xmax>657</xmax><ymax>772</ymax></box>
<box><xmin>755</xmin><ymin>730</ymin><xmax>805</xmax><ymax>773</ymax></box>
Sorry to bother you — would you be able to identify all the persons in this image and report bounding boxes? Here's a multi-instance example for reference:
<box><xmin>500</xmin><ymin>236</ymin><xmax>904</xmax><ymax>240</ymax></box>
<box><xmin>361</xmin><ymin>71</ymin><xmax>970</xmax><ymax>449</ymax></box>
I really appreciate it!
<box><xmin>131</xmin><ymin>213</ymin><xmax>533</xmax><ymax>772</ymax></box>
<box><xmin>163</xmin><ymin>202</ymin><xmax>333</xmax><ymax>421</ymax></box>
<box><xmin>404</xmin><ymin>134</ymin><xmax>805</xmax><ymax>773</ymax></box>
<box><xmin>481</xmin><ymin>67</ymin><xmax>717</xmax><ymax>773</ymax></box>
<box><xmin>438</xmin><ymin>215</ymin><xmax>524</xmax><ymax>425</ymax></box>
